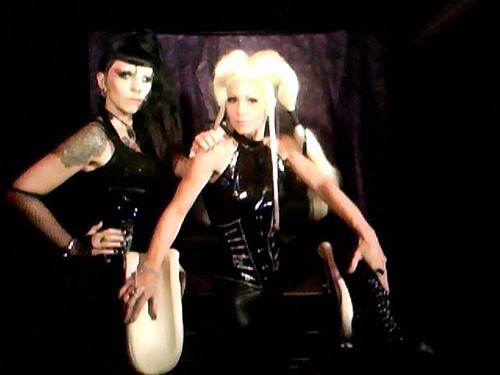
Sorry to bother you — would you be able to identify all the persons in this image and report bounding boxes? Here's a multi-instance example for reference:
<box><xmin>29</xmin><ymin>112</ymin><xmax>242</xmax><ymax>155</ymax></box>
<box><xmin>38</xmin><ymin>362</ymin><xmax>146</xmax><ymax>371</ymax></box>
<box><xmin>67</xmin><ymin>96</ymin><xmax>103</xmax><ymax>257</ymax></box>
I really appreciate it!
<box><xmin>119</xmin><ymin>50</ymin><xmax>391</xmax><ymax>374</ymax></box>
<box><xmin>9</xmin><ymin>38</ymin><xmax>207</xmax><ymax>375</ymax></box>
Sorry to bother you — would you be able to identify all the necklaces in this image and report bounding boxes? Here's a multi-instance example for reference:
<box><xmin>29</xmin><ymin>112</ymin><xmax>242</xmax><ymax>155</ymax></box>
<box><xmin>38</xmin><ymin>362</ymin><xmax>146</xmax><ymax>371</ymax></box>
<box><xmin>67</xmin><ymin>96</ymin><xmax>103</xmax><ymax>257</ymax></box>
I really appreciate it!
<box><xmin>105</xmin><ymin>106</ymin><xmax>137</xmax><ymax>139</ymax></box>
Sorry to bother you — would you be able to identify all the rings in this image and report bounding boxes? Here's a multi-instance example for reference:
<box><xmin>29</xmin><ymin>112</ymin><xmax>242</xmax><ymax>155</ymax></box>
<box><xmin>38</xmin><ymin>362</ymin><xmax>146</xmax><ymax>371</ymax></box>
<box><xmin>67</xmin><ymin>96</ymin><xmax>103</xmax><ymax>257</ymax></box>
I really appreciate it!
<box><xmin>375</xmin><ymin>268</ymin><xmax>384</xmax><ymax>276</ymax></box>
<box><xmin>131</xmin><ymin>290</ymin><xmax>138</xmax><ymax>299</ymax></box>
<box><xmin>100</xmin><ymin>235</ymin><xmax>106</xmax><ymax>243</ymax></box>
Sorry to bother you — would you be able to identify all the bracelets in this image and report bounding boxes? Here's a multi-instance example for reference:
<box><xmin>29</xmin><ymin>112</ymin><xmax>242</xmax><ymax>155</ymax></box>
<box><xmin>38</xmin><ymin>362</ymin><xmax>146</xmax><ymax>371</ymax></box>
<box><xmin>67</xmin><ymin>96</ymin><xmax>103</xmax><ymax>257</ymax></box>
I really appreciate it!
<box><xmin>62</xmin><ymin>238</ymin><xmax>76</xmax><ymax>257</ymax></box>
